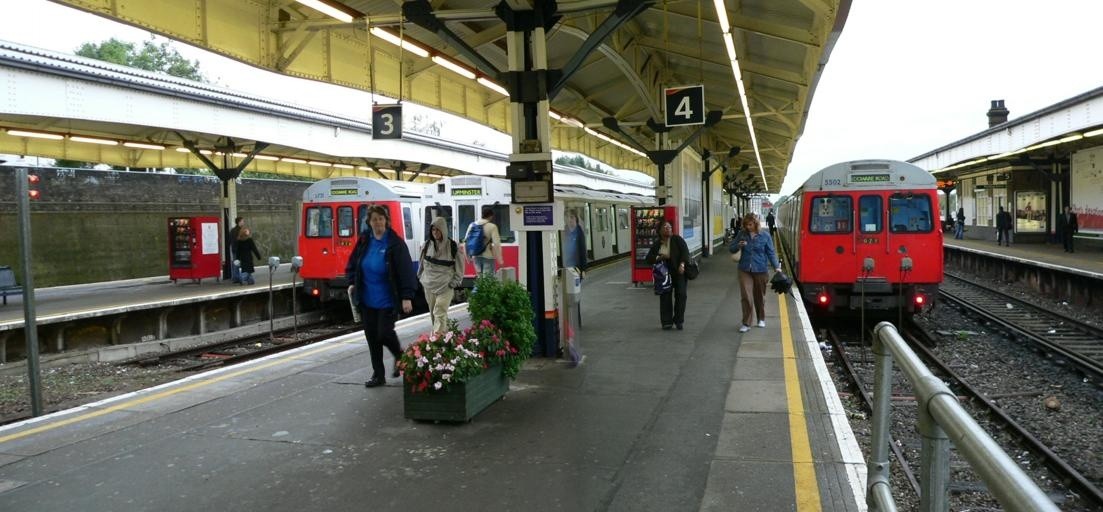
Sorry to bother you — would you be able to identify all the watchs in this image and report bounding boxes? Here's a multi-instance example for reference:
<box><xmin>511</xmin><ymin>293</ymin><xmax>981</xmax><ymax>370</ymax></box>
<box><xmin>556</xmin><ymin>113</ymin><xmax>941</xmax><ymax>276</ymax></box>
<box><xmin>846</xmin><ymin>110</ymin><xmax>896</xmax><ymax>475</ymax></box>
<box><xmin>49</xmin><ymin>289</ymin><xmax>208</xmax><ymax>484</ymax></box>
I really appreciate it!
<box><xmin>680</xmin><ymin>264</ymin><xmax>684</xmax><ymax>268</ymax></box>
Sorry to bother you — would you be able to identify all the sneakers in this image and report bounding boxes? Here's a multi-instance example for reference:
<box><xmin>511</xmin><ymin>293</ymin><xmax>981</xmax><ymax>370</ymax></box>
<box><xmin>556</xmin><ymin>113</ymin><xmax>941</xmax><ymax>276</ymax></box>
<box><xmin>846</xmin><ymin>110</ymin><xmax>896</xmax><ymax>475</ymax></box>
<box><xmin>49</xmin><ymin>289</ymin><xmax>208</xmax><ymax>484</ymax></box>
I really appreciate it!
<box><xmin>391</xmin><ymin>357</ymin><xmax>402</xmax><ymax>378</ymax></box>
<box><xmin>675</xmin><ymin>322</ymin><xmax>682</xmax><ymax>330</ymax></box>
<box><xmin>738</xmin><ymin>324</ymin><xmax>751</xmax><ymax>334</ymax></box>
<box><xmin>757</xmin><ymin>320</ymin><xmax>765</xmax><ymax>327</ymax></box>
<box><xmin>365</xmin><ymin>376</ymin><xmax>385</xmax><ymax>387</ymax></box>
<box><xmin>662</xmin><ymin>324</ymin><xmax>672</xmax><ymax>330</ymax></box>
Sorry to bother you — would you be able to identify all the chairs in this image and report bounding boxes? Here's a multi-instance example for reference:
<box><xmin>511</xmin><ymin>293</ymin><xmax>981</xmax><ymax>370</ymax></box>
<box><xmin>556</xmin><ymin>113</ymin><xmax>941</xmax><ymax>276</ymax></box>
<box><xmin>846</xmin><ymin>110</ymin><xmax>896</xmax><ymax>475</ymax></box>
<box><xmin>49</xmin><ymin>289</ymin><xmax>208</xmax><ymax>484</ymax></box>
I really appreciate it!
<box><xmin>0</xmin><ymin>267</ymin><xmax>23</xmax><ymax>305</ymax></box>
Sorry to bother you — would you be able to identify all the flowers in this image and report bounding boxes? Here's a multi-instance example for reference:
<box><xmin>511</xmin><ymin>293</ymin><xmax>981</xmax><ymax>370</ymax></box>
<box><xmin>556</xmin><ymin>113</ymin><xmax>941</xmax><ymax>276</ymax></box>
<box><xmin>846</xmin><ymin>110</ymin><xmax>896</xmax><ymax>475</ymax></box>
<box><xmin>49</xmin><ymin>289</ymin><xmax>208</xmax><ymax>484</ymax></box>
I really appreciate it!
<box><xmin>390</xmin><ymin>318</ymin><xmax>518</xmax><ymax>399</ymax></box>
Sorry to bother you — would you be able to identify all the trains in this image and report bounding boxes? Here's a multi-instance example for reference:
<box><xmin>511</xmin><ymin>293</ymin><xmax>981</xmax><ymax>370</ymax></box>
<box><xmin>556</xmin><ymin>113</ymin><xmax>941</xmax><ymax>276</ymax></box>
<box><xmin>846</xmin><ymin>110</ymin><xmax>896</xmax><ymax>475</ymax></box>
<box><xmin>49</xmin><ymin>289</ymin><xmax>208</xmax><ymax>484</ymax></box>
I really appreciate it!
<box><xmin>771</xmin><ymin>157</ymin><xmax>945</xmax><ymax>330</ymax></box>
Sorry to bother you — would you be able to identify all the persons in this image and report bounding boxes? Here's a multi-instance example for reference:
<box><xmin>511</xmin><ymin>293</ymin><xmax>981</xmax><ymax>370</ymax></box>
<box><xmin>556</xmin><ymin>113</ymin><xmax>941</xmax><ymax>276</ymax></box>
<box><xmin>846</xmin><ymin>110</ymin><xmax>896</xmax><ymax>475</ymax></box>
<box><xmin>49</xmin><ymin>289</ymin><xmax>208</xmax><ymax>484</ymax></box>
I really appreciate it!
<box><xmin>464</xmin><ymin>209</ymin><xmax>505</xmax><ymax>292</ymax></box>
<box><xmin>344</xmin><ymin>203</ymin><xmax>414</xmax><ymax>388</ymax></box>
<box><xmin>729</xmin><ymin>212</ymin><xmax>781</xmax><ymax>334</ymax></box>
<box><xmin>953</xmin><ymin>208</ymin><xmax>966</xmax><ymax>241</ymax></box>
<box><xmin>236</xmin><ymin>226</ymin><xmax>259</xmax><ymax>285</ymax></box>
<box><xmin>227</xmin><ymin>217</ymin><xmax>242</xmax><ymax>282</ymax></box>
<box><xmin>646</xmin><ymin>219</ymin><xmax>692</xmax><ymax>330</ymax></box>
<box><xmin>737</xmin><ymin>216</ymin><xmax>741</xmax><ymax>236</ymax></box>
<box><xmin>416</xmin><ymin>216</ymin><xmax>463</xmax><ymax>338</ymax></box>
<box><xmin>730</xmin><ymin>218</ymin><xmax>736</xmax><ymax>239</ymax></box>
<box><xmin>563</xmin><ymin>208</ymin><xmax>587</xmax><ymax>281</ymax></box>
<box><xmin>1024</xmin><ymin>202</ymin><xmax>1032</xmax><ymax>222</ymax></box>
<box><xmin>766</xmin><ymin>211</ymin><xmax>777</xmax><ymax>235</ymax></box>
<box><xmin>1035</xmin><ymin>210</ymin><xmax>1046</xmax><ymax>228</ymax></box>
<box><xmin>996</xmin><ymin>206</ymin><xmax>1011</xmax><ymax>247</ymax></box>
<box><xmin>945</xmin><ymin>215</ymin><xmax>953</xmax><ymax>232</ymax></box>
<box><xmin>1058</xmin><ymin>206</ymin><xmax>1077</xmax><ymax>254</ymax></box>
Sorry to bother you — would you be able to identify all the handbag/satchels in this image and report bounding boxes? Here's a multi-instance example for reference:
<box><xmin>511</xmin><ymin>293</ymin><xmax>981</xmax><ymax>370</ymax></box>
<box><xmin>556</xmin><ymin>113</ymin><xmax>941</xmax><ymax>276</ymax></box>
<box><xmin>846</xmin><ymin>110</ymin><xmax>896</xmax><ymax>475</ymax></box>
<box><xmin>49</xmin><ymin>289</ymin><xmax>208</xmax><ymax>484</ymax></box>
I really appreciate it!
<box><xmin>652</xmin><ymin>255</ymin><xmax>673</xmax><ymax>295</ymax></box>
<box><xmin>346</xmin><ymin>285</ymin><xmax>362</xmax><ymax>323</ymax></box>
<box><xmin>685</xmin><ymin>258</ymin><xmax>699</xmax><ymax>280</ymax></box>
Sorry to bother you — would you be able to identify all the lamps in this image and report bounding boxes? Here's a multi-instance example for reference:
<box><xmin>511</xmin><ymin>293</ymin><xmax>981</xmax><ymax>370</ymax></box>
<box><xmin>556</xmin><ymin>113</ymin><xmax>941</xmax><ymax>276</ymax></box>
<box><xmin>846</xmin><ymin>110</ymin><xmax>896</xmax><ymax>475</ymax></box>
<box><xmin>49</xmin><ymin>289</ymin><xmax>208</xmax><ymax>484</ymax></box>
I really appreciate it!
<box><xmin>549</xmin><ymin>111</ymin><xmax>651</xmax><ymax>160</ymax></box>
<box><xmin>68</xmin><ymin>135</ymin><xmax>120</xmax><ymax>145</ymax></box>
<box><xmin>370</xmin><ymin>26</ymin><xmax>429</xmax><ymax>57</ymax></box>
<box><xmin>478</xmin><ymin>76</ymin><xmax>510</xmax><ymax>98</ymax></box>
<box><xmin>432</xmin><ymin>56</ymin><xmax>477</xmax><ymax>80</ymax></box>
<box><xmin>298</xmin><ymin>1</ymin><xmax>354</xmax><ymax>24</ymax></box>
<box><xmin>123</xmin><ymin>142</ymin><xmax>166</xmax><ymax>150</ymax></box>
<box><xmin>6</xmin><ymin>128</ymin><xmax>66</xmax><ymax>140</ymax></box>
<box><xmin>175</xmin><ymin>146</ymin><xmax>456</xmax><ymax>180</ymax></box>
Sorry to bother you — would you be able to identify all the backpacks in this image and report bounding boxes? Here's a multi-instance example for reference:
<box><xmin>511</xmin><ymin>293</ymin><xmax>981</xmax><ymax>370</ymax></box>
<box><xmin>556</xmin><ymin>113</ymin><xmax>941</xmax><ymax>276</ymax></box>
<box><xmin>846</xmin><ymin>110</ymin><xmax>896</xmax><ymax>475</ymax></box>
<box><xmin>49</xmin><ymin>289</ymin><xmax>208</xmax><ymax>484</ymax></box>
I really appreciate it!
<box><xmin>463</xmin><ymin>221</ymin><xmax>489</xmax><ymax>256</ymax></box>
